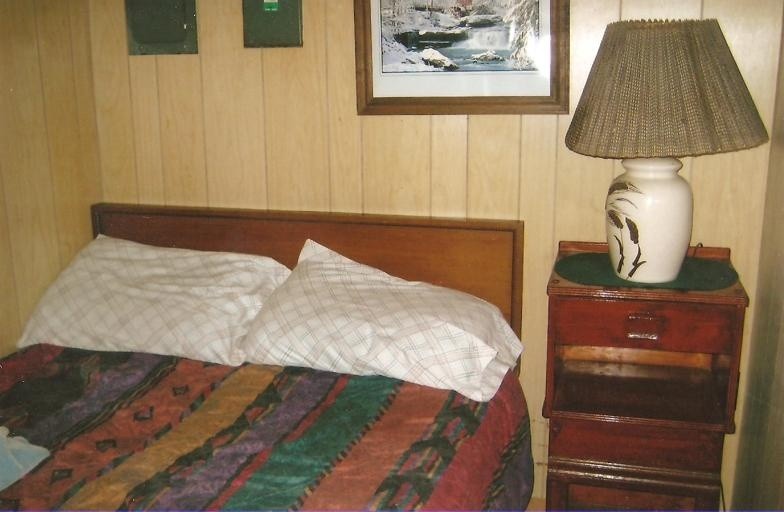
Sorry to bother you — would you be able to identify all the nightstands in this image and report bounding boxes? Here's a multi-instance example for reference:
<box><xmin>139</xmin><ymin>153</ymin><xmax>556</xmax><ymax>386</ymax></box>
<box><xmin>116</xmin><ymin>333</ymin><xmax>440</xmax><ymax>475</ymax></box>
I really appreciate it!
<box><xmin>541</xmin><ymin>241</ymin><xmax>750</xmax><ymax>512</ymax></box>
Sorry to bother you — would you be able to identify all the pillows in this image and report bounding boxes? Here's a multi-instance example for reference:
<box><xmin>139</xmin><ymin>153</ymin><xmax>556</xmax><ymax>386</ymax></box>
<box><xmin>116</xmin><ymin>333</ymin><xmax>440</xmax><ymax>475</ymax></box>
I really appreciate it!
<box><xmin>17</xmin><ymin>231</ymin><xmax>291</xmax><ymax>369</ymax></box>
<box><xmin>239</xmin><ymin>237</ymin><xmax>523</xmax><ymax>402</ymax></box>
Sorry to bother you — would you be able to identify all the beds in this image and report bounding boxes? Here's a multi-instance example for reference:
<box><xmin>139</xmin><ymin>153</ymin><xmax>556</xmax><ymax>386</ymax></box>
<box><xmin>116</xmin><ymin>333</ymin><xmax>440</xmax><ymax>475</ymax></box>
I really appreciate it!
<box><xmin>1</xmin><ymin>202</ymin><xmax>533</xmax><ymax>511</ymax></box>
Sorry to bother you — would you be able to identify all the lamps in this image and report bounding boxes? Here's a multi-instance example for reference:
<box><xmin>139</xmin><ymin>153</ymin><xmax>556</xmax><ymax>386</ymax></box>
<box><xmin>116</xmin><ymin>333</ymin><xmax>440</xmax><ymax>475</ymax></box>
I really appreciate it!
<box><xmin>562</xmin><ymin>16</ymin><xmax>770</xmax><ymax>285</ymax></box>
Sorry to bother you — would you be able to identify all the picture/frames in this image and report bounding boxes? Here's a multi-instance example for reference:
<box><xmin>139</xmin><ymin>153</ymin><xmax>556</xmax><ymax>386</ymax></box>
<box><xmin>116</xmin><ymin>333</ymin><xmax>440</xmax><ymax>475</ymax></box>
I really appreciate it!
<box><xmin>353</xmin><ymin>0</ymin><xmax>570</xmax><ymax>114</ymax></box>
